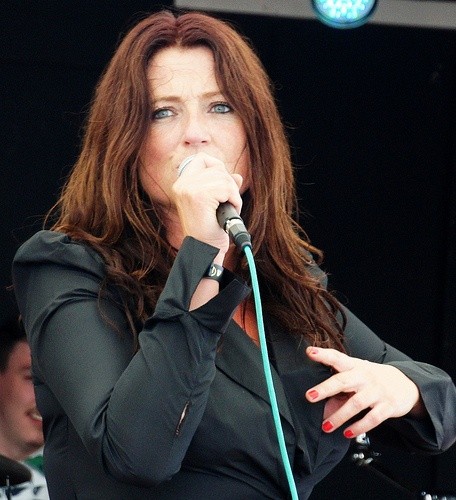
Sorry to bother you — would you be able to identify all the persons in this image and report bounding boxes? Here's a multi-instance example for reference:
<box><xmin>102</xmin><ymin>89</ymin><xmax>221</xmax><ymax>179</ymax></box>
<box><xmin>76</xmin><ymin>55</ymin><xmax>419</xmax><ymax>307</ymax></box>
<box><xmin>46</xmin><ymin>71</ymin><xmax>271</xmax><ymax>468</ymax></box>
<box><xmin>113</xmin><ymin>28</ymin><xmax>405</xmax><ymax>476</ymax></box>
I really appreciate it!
<box><xmin>0</xmin><ymin>324</ymin><xmax>51</xmax><ymax>500</ymax></box>
<box><xmin>11</xmin><ymin>11</ymin><xmax>456</xmax><ymax>500</ymax></box>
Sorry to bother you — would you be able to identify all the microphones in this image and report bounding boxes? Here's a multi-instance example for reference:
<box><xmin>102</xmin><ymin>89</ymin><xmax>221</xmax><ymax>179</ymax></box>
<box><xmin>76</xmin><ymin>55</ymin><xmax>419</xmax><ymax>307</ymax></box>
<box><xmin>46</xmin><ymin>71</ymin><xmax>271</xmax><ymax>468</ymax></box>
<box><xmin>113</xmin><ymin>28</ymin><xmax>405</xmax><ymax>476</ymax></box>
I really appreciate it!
<box><xmin>178</xmin><ymin>155</ymin><xmax>251</xmax><ymax>251</ymax></box>
